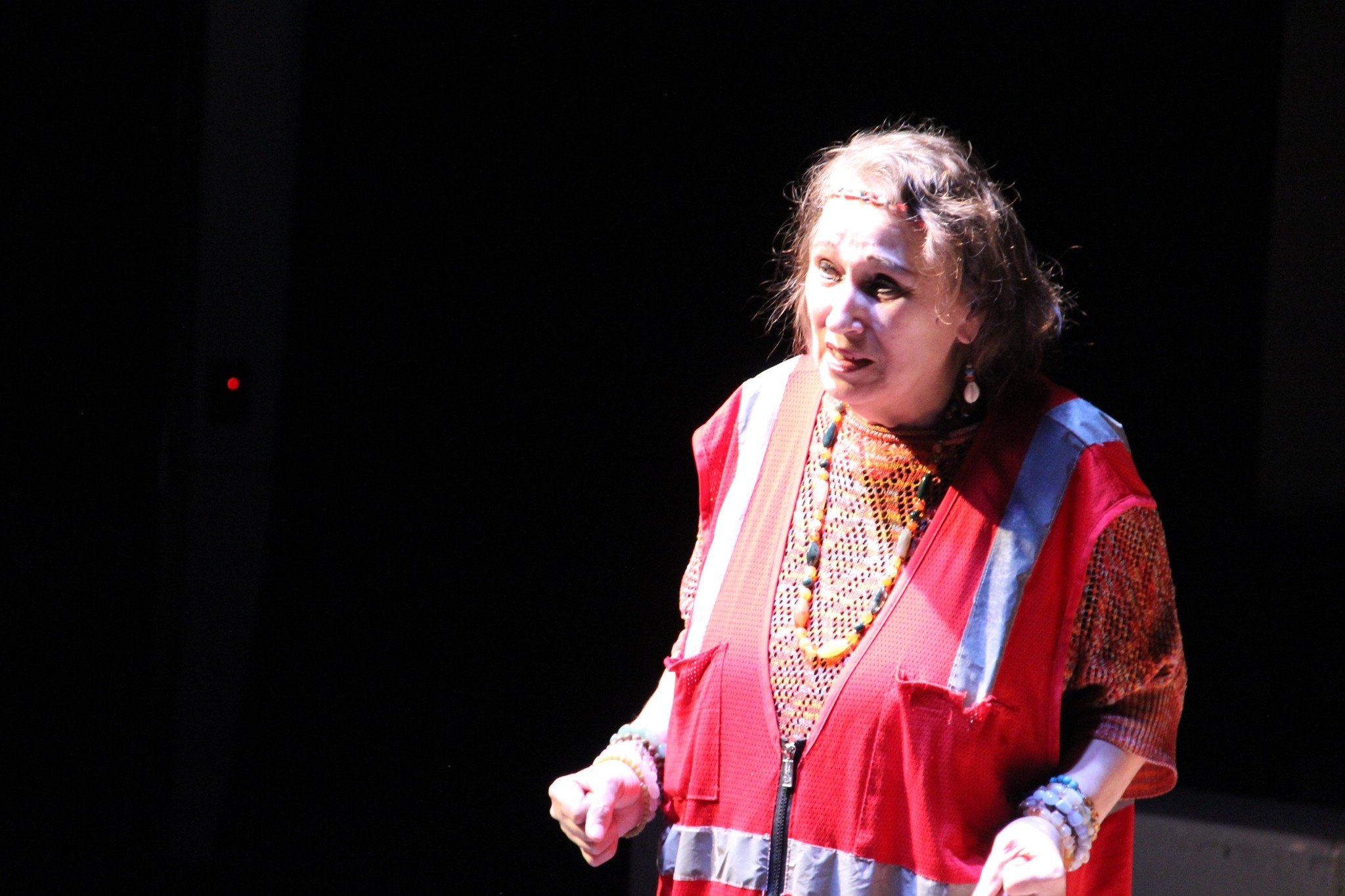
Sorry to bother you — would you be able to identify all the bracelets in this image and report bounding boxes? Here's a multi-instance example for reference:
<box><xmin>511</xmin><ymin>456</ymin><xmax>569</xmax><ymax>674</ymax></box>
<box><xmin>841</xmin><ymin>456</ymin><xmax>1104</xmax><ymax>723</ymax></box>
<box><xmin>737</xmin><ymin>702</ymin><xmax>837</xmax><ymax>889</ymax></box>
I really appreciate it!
<box><xmin>1019</xmin><ymin>774</ymin><xmax>1102</xmax><ymax>873</ymax></box>
<box><xmin>592</xmin><ymin>723</ymin><xmax>671</xmax><ymax>837</ymax></box>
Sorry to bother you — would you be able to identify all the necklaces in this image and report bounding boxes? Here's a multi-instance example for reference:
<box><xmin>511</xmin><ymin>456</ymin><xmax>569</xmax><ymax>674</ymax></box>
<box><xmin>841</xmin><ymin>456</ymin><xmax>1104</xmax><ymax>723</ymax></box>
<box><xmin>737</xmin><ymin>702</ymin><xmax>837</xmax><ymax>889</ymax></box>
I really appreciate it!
<box><xmin>795</xmin><ymin>400</ymin><xmax>960</xmax><ymax>664</ymax></box>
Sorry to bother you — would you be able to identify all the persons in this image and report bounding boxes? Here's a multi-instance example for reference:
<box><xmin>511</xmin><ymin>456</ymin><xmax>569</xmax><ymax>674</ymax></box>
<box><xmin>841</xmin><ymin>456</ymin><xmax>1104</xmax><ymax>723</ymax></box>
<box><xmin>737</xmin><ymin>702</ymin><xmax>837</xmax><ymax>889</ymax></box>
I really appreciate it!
<box><xmin>547</xmin><ymin>133</ymin><xmax>1186</xmax><ymax>896</ymax></box>
